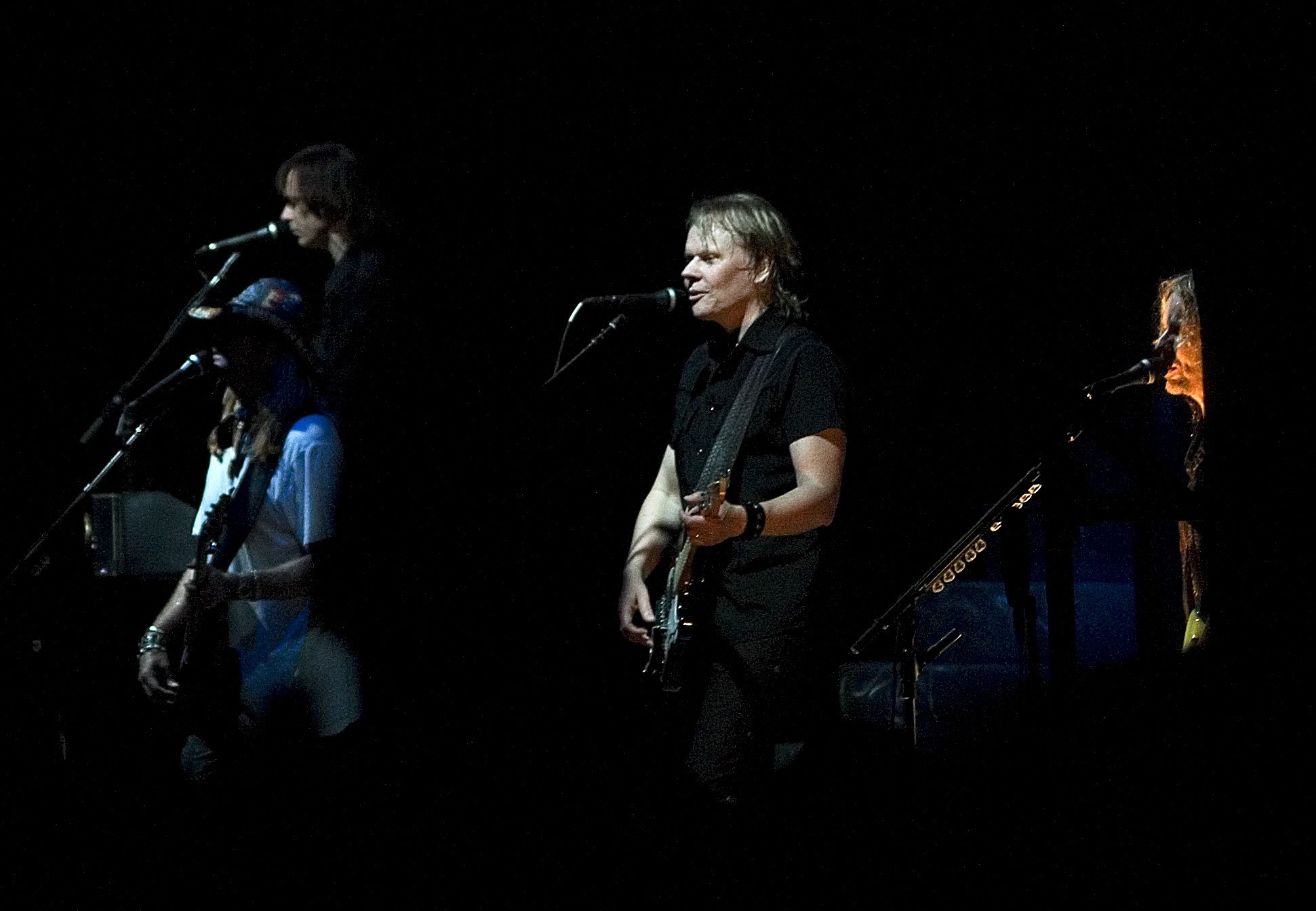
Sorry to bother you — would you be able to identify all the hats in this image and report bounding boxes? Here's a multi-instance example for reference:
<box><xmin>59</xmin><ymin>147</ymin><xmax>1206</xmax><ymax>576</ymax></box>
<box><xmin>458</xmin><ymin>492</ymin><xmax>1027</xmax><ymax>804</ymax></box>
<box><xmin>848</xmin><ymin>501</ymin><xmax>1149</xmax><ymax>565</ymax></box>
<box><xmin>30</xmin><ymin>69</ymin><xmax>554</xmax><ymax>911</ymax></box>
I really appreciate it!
<box><xmin>228</xmin><ymin>278</ymin><xmax>306</xmax><ymax>335</ymax></box>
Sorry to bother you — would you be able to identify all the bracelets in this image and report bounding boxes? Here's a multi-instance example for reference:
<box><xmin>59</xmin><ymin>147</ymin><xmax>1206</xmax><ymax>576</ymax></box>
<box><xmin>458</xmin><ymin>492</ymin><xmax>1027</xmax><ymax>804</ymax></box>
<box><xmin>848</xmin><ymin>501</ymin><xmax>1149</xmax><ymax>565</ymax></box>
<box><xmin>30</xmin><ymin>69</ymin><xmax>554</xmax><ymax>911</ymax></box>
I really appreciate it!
<box><xmin>137</xmin><ymin>629</ymin><xmax>170</xmax><ymax>656</ymax></box>
<box><xmin>736</xmin><ymin>501</ymin><xmax>765</xmax><ymax>542</ymax></box>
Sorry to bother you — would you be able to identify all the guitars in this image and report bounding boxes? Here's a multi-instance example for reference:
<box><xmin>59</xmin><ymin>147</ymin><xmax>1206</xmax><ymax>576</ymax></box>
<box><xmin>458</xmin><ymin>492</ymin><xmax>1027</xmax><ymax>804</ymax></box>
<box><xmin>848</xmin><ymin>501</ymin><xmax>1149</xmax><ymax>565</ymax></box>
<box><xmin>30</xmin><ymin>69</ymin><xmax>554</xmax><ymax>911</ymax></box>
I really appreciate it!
<box><xmin>160</xmin><ymin>494</ymin><xmax>231</xmax><ymax>703</ymax></box>
<box><xmin>191</xmin><ymin>394</ymin><xmax>245</xmax><ymax>538</ymax></box>
<box><xmin>641</xmin><ymin>462</ymin><xmax>732</xmax><ymax>693</ymax></box>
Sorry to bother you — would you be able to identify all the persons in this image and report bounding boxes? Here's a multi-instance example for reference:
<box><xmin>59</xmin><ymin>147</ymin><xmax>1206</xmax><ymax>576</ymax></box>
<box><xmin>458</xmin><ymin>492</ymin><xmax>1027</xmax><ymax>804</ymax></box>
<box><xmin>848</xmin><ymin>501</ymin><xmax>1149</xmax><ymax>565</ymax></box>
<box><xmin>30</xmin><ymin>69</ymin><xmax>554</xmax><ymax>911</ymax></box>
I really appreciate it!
<box><xmin>135</xmin><ymin>141</ymin><xmax>404</xmax><ymax>784</ymax></box>
<box><xmin>617</xmin><ymin>192</ymin><xmax>847</xmax><ymax>808</ymax></box>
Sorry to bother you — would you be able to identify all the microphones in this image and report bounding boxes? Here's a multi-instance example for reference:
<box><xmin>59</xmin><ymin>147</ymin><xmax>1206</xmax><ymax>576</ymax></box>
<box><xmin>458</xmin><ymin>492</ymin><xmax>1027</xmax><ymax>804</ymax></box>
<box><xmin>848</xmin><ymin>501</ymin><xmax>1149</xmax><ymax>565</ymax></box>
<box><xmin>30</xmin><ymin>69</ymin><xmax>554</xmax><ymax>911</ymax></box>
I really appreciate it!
<box><xmin>583</xmin><ymin>288</ymin><xmax>687</xmax><ymax>311</ymax></box>
<box><xmin>126</xmin><ymin>350</ymin><xmax>211</xmax><ymax>412</ymax></box>
<box><xmin>1079</xmin><ymin>357</ymin><xmax>1169</xmax><ymax>399</ymax></box>
<box><xmin>195</xmin><ymin>222</ymin><xmax>286</xmax><ymax>257</ymax></box>
